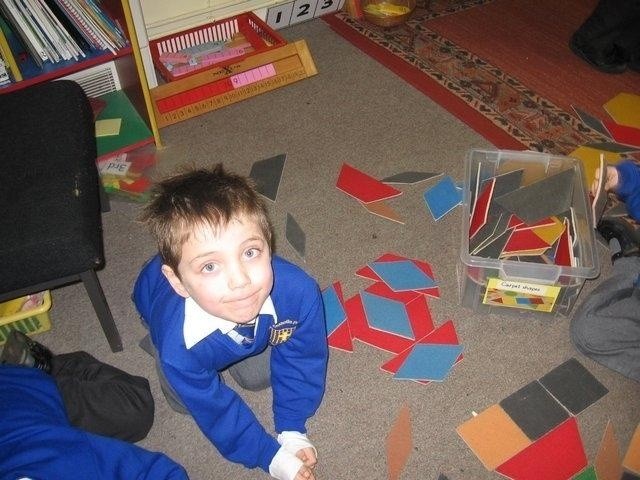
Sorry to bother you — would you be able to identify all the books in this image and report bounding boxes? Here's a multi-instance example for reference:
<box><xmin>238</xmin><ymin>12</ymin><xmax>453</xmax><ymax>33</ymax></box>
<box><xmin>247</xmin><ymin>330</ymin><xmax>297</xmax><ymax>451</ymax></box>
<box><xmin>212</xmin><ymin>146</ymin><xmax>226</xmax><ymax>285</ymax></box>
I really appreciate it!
<box><xmin>1</xmin><ymin>0</ymin><xmax>129</xmax><ymax>89</ymax></box>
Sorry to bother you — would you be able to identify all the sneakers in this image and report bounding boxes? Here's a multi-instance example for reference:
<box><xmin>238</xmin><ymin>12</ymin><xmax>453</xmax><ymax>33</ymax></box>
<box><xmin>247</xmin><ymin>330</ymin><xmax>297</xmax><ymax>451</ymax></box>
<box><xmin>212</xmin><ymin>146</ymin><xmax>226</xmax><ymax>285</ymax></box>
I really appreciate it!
<box><xmin>2</xmin><ymin>331</ymin><xmax>53</xmax><ymax>374</ymax></box>
<box><xmin>597</xmin><ymin>214</ymin><xmax>640</xmax><ymax>263</ymax></box>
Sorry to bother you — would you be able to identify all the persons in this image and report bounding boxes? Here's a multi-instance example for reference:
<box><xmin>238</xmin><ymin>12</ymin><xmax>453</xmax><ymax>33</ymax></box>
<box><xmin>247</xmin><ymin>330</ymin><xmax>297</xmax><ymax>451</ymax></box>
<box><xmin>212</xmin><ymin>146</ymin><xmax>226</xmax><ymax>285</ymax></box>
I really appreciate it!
<box><xmin>570</xmin><ymin>160</ymin><xmax>638</xmax><ymax>384</ymax></box>
<box><xmin>0</xmin><ymin>328</ymin><xmax>189</xmax><ymax>479</ymax></box>
<box><xmin>131</xmin><ymin>163</ymin><xmax>328</xmax><ymax>480</ymax></box>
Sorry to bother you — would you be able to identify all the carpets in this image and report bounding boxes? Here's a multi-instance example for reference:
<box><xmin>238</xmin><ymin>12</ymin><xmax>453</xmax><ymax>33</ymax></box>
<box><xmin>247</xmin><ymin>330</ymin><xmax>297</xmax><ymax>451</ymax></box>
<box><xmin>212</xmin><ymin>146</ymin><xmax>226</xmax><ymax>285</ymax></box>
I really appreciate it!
<box><xmin>322</xmin><ymin>1</ymin><xmax>640</xmax><ymax>232</ymax></box>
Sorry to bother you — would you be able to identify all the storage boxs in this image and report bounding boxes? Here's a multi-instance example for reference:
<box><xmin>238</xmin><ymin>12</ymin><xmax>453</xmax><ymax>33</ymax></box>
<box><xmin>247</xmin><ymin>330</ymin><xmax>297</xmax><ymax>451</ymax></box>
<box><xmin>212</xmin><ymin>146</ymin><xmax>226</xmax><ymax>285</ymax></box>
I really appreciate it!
<box><xmin>454</xmin><ymin>148</ymin><xmax>602</xmax><ymax>318</ymax></box>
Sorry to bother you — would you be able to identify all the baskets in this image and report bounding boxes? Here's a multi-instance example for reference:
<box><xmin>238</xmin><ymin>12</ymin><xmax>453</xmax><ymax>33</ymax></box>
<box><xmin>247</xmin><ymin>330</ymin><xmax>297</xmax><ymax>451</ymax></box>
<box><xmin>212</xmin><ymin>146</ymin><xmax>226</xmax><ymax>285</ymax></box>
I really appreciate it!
<box><xmin>0</xmin><ymin>289</ymin><xmax>51</xmax><ymax>346</ymax></box>
<box><xmin>149</xmin><ymin>11</ymin><xmax>287</xmax><ymax>84</ymax></box>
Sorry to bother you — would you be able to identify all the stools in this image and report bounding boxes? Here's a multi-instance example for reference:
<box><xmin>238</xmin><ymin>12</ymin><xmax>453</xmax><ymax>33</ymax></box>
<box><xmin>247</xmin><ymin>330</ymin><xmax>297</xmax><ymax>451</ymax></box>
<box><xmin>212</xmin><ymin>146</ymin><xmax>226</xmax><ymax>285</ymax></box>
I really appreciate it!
<box><xmin>1</xmin><ymin>76</ymin><xmax>127</xmax><ymax>360</ymax></box>
